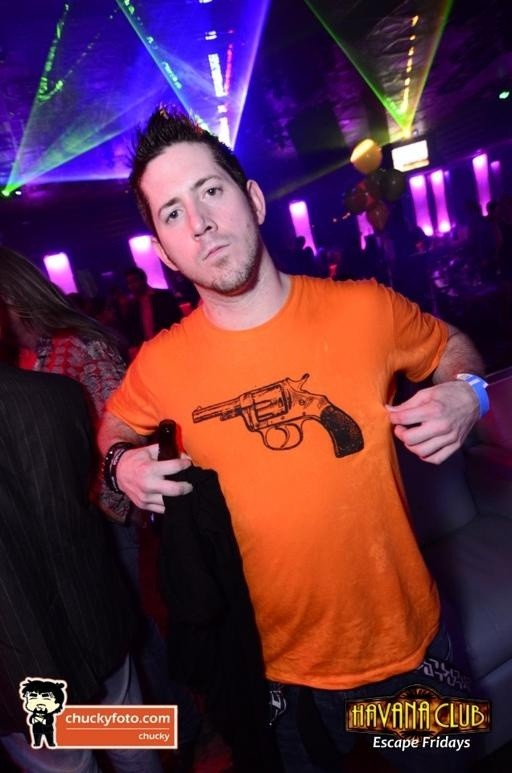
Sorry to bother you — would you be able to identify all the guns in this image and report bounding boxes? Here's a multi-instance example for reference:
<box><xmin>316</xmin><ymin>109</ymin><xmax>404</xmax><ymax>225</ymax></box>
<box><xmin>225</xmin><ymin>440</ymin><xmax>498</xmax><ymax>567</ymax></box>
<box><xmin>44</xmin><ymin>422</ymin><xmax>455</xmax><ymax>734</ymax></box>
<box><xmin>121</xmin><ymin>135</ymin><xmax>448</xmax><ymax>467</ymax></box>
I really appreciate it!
<box><xmin>189</xmin><ymin>372</ymin><xmax>365</xmax><ymax>458</ymax></box>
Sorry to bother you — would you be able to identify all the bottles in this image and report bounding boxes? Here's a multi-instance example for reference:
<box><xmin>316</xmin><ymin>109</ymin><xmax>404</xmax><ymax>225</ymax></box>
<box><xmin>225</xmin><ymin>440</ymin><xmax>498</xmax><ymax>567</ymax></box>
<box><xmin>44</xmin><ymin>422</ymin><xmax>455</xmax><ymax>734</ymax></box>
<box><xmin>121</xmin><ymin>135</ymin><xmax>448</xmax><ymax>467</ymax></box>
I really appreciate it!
<box><xmin>150</xmin><ymin>417</ymin><xmax>185</xmax><ymax>533</ymax></box>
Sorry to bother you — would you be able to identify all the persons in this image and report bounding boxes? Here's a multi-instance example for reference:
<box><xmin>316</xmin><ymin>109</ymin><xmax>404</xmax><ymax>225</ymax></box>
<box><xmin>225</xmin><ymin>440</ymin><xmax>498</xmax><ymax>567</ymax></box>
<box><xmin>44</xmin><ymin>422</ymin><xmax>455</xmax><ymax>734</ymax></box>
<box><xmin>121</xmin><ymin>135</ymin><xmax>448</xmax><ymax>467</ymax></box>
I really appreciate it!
<box><xmin>0</xmin><ymin>243</ymin><xmax>208</xmax><ymax>771</ymax></box>
<box><xmin>66</xmin><ymin>196</ymin><xmax>511</xmax><ymax>339</ymax></box>
<box><xmin>92</xmin><ymin>103</ymin><xmax>489</xmax><ymax>771</ymax></box>
<box><xmin>0</xmin><ymin>362</ymin><xmax>163</xmax><ymax>771</ymax></box>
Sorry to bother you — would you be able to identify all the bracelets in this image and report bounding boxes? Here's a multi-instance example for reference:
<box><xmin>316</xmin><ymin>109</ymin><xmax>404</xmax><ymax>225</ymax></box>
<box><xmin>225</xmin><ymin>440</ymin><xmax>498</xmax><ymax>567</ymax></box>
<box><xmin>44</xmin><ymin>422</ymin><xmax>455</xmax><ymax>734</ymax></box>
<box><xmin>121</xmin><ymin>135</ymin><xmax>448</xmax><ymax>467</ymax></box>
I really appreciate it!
<box><xmin>454</xmin><ymin>370</ymin><xmax>490</xmax><ymax>417</ymax></box>
<box><xmin>100</xmin><ymin>440</ymin><xmax>133</xmax><ymax>496</ymax></box>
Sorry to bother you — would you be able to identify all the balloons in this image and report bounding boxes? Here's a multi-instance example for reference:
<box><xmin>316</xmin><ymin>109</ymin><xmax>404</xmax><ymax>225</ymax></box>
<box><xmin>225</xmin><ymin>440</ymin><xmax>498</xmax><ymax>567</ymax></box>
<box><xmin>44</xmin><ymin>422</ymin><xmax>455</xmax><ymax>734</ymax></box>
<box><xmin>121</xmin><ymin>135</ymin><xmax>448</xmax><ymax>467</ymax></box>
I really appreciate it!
<box><xmin>342</xmin><ymin>132</ymin><xmax>406</xmax><ymax>232</ymax></box>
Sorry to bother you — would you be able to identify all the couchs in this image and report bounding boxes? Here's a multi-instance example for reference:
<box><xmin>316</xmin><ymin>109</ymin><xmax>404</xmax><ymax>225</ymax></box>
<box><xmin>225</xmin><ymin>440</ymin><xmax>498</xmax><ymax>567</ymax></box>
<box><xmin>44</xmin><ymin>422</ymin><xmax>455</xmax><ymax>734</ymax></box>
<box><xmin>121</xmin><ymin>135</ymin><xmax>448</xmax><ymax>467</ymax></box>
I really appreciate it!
<box><xmin>397</xmin><ymin>438</ymin><xmax>511</xmax><ymax>770</ymax></box>
